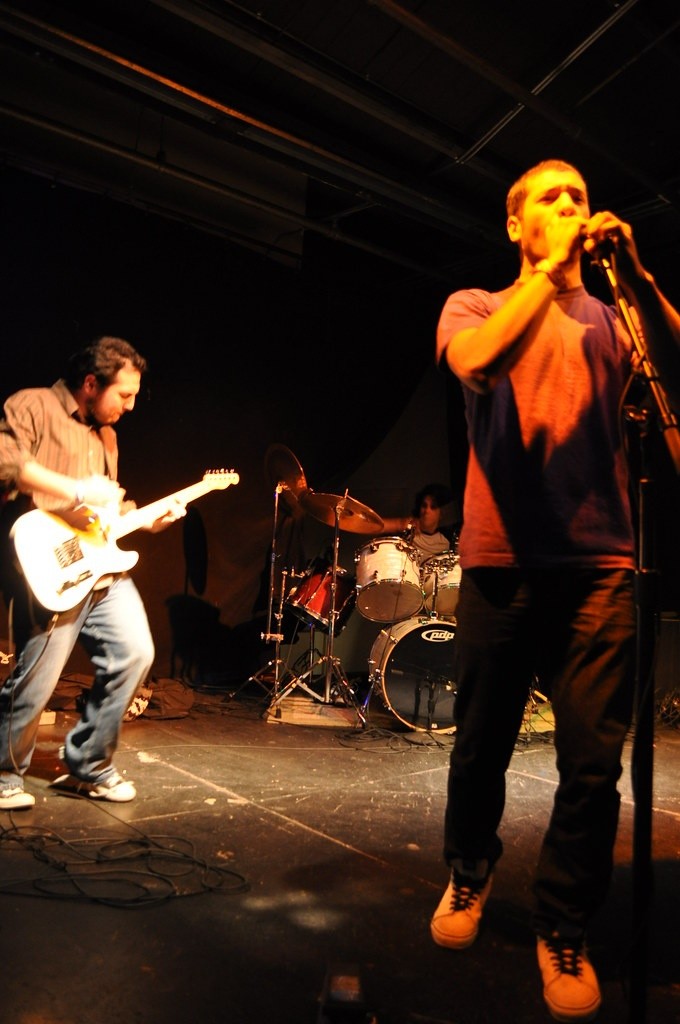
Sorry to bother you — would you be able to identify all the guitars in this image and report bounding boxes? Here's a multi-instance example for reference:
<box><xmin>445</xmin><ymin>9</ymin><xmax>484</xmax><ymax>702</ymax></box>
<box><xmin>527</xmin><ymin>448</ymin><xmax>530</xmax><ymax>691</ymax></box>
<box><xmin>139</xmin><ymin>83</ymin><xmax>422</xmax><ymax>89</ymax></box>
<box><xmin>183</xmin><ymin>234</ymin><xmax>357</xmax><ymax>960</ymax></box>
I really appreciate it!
<box><xmin>8</xmin><ymin>465</ymin><xmax>243</xmax><ymax>619</ymax></box>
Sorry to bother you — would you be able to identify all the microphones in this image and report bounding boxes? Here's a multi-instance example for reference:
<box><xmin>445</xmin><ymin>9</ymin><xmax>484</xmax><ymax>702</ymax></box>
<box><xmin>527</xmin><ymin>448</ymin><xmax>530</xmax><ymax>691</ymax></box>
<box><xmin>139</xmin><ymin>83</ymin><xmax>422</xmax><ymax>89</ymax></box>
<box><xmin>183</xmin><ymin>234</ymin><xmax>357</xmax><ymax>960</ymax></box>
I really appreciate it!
<box><xmin>580</xmin><ymin>227</ymin><xmax>618</xmax><ymax>249</ymax></box>
<box><xmin>402</xmin><ymin>521</ymin><xmax>412</xmax><ymax>538</ymax></box>
<box><xmin>449</xmin><ymin>533</ymin><xmax>456</xmax><ymax>553</ymax></box>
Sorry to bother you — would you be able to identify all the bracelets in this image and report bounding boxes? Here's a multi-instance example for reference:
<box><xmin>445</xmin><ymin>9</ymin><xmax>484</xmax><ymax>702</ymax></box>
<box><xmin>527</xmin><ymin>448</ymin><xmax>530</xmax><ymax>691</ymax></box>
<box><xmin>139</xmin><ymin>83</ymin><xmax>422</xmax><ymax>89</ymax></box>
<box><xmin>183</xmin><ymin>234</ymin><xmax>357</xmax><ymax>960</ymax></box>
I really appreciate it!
<box><xmin>531</xmin><ymin>259</ymin><xmax>569</xmax><ymax>288</ymax></box>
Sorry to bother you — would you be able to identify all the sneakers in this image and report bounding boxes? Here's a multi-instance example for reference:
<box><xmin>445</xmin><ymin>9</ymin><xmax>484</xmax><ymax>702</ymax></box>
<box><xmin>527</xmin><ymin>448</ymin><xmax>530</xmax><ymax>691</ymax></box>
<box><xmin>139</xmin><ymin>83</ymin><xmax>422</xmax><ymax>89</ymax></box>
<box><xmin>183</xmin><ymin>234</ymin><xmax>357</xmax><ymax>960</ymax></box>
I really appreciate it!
<box><xmin>534</xmin><ymin>924</ymin><xmax>600</xmax><ymax>1018</ymax></box>
<box><xmin>59</xmin><ymin>745</ymin><xmax>136</xmax><ymax>802</ymax></box>
<box><xmin>431</xmin><ymin>867</ymin><xmax>492</xmax><ymax>948</ymax></box>
<box><xmin>0</xmin><ymin>785</ymin><xmax>34</xmax><ymax>808</ymax></box>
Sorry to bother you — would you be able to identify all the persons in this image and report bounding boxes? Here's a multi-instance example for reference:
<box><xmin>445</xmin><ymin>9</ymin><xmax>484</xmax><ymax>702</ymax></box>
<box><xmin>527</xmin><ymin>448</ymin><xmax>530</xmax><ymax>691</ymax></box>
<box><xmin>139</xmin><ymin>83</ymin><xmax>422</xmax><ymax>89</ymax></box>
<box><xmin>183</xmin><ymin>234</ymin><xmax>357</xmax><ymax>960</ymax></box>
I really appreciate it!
<box><xmin>430</xmin><ymin>159</ymin><xmax>680</xmax><ymax>1021</ymax></box>
<box><xmin>0</xmin><ymin>337</ymin><xmax>188</xmax><ymax>809</ymax></box>
<box><xmin>404</xmin><ymin>486</ymin><xmax>459</xmax><ymax>565</ymax></box>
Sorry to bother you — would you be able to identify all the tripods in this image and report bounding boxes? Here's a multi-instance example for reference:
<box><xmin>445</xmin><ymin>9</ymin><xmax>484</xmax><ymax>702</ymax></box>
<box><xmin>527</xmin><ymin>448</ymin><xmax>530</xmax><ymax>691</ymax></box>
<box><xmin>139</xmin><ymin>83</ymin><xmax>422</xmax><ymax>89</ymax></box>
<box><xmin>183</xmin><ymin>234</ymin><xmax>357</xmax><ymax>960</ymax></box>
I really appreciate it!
<box><xmin>223</xmin><ymin>482</ymin><xmax>370</xmax><ymax>730</ymax></box>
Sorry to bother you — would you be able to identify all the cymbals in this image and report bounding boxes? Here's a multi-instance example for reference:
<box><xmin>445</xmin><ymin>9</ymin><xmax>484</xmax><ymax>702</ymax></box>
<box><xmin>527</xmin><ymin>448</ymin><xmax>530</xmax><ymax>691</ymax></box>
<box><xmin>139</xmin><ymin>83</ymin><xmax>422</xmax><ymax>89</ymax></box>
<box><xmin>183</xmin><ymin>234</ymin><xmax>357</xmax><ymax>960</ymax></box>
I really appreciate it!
<box><xmin>297</xmin><ymin>489</ymin><xmax>384</xmax><ymax>536</ymax></box>
<box><xmin>262</xmin><ymin>442</ymin><xmax>308</xmax><ymax>520</ymax></box>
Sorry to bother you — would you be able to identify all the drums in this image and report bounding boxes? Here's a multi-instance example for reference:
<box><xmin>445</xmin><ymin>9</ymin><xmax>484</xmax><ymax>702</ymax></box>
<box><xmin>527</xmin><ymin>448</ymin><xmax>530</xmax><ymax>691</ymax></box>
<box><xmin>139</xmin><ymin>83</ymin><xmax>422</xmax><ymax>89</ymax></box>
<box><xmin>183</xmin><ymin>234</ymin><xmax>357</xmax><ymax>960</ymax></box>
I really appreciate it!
<box><xmin>367</xmin><ymin>614</ymin><xmax>457</xmax><ymax>725</ymax></box>
<box><xmin>350</xmin><ymin>536</ymin><xmax>425</xmax><ymax>623</ymax></box>
<box><xmin>282</xmin><ymin>554</ymin><xmax>357</xmax><ymax>639</ymax></box>
<box><xmin>423</xmin><ymin>549</ymin><xmax>462</xmax><ymax>620</ymax></box>
